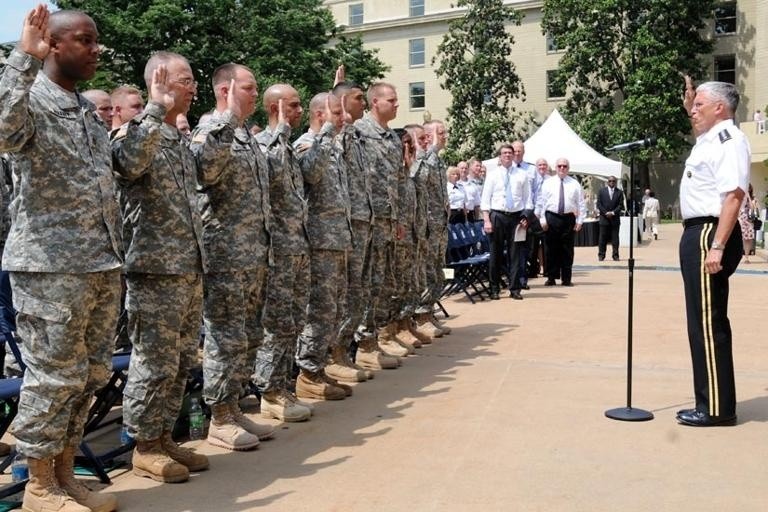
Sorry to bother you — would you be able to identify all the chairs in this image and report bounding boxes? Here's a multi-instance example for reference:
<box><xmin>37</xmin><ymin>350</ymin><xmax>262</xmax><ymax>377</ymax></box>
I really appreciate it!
<box><xmin>439</xmin><ymin>221</ymin><xmax>508</xmax><ymax>304</ymax></box>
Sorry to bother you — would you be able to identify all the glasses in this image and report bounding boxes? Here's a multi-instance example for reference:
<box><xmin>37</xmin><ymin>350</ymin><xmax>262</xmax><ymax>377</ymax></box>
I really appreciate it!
<box><xmin>170</xmin><ymin>78</ymin><xmax>199</xmax><ymax>87</ymax></box>
<box><xmin>558</xmin><ymin>165</ymin><xmax>568</xmax><ymax>167</ymax></box>
<box><xmin>609</xmin><ymin>180</ymin><xmax>615</xmax><ymax>182</ymax></box>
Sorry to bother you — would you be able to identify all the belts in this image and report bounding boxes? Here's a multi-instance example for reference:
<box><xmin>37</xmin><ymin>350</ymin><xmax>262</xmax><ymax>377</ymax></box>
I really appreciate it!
<box><xmin>546</xmin><ymin>211</ymin><xmax>574</xmax><ymax>217</ymax></box>
<box><xmin>493</xmin><ymin>210</ymin><xmax>520</xmax><ymax>216</ymax></box>
<box><xmin>681</xmin><ymin>216</ymin><xmax>718</xmax><ymax>226</ymax></box>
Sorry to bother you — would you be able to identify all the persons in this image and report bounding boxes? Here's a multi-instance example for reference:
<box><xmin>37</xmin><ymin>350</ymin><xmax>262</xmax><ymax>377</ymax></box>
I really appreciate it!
<box><xmin>508</xmin><ymin>139</ymin><xmax>539</xmax><ymax>290</ymax></box>
<box><xmin>738</xmin><ymin>184</ymin><xmax>756</xmax><ymax>256</ymax></box>
<box><xmin>755</xmin><ymin>109</ymin><xmax>760</xmax><ymax>121</ymax></box>
<box><xmin>533</xmin><ymin>158</ymin><xmax>552</xmax><ymax>278</ymax></box>
<box><xmin>643</xmin><ymin>192</ymin><xmax>660</xmax><ymax>241</ymax></box>
<box><xmin>480</xmin><ymin>144</ymin><xmax>530</xmax><ymax>301</ymax></box>
<box><xmin>444</xmin><ymin>157</ymin><xmax>487</xmax><ymax>225</ymax></box>
<box><xmin>1</xmin><ymin>4</ymin><xmax>451</xmax><ymax>512</ymax></box>
<box><xmin>675</xmin><ymin>77</ymin><xmax>752</xmax><ymax>427</ymax></box>
<box><xmin>642</xmin><ymin>189</ymin><xmax>650</xmax><ymax>234</ymax></box>
<box><xmin>597</xmin><ymin>176</ymin><xmax>623</xmax><ymax>261</ymax></box>
<box><xmin>542</xmin><ymin>156</ymin><xmax>586</xmax><ymax>287</ymax></box>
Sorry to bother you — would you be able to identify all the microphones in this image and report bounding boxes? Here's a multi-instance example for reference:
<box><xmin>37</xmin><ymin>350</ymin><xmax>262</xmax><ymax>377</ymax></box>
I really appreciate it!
<box><xmin>604</xmin><ymin>136</ymin><xmax>658</xmax><ymax>154</ymax></box>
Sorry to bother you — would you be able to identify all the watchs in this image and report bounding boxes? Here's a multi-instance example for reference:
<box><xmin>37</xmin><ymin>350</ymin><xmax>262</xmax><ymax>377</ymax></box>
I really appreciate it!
<box><xmin>710</xmin><ymin>242</ymin><xmax>727</xmax><ymax>250</ymax></box>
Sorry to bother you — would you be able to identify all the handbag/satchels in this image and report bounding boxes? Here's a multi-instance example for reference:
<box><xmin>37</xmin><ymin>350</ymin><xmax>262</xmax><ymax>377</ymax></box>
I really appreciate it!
<box><xmin>755</xmin><ymin>220</ymin><xmax>761</xmax><ymax>229</ymax></box>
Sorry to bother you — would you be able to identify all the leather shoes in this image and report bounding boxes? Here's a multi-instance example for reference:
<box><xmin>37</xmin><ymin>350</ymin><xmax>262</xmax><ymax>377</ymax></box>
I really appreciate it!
<box><xmin>490</xmin><ymin>285</ymin><xmax>530</xmax><ymax>300</ymax></box>
<box><xmin>599</xmin><ymin>256</ymin><xmax>619</xmax><ymax>261</ymax></box>
<box><xmin>677</xmin><ymin>409</ymin><xmax>736</xmax><ymax>426</ymax></box>
<box><xmin>545</xmin><ymin>280</ymin><xmax>556</xmax><ymax>285</ymax></box>
<box><xmin>562</xmin><ymin>281</ymin><xmax>573</xmax><ymax>286</ymax></box>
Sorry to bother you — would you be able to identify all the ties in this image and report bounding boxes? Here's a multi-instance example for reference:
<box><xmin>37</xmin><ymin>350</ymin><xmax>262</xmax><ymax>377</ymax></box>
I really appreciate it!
<box><xmin>504</xmin><ymin>169</ymin><xmax>513</xmax><ymax>209</ymax></box>
<box><xmin>559</xmin><ymin>178</ymin><xmax>564</xmax><ymax>216</ymax></box>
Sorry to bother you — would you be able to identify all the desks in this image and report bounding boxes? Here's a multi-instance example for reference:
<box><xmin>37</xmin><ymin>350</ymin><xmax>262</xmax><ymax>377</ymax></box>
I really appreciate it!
<box><xmin>573</xmin><ymin>218</ymin><xmax>600</xmax><ymax>247</ymax></box>
